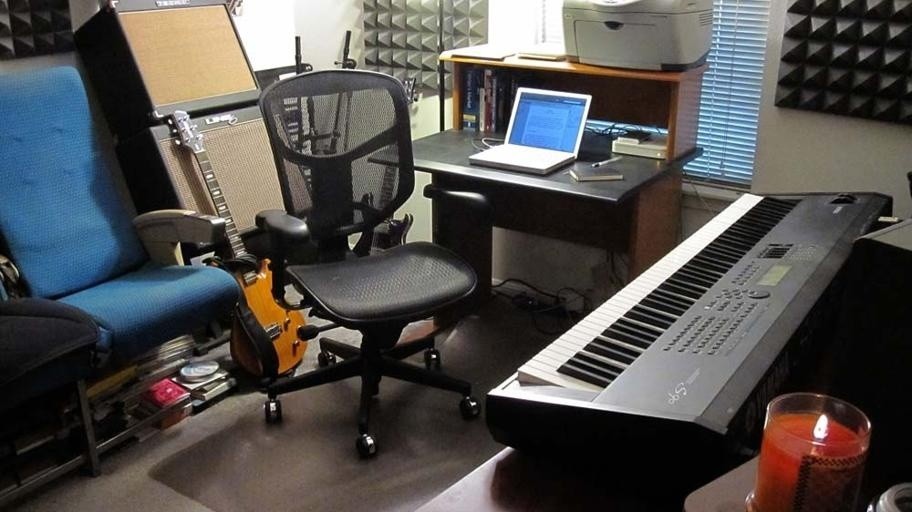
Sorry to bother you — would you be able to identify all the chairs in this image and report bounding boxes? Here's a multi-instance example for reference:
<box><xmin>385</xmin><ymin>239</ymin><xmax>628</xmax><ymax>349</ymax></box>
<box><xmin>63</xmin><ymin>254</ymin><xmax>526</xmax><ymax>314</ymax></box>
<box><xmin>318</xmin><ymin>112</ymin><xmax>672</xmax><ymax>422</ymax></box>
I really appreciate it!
<box><xmin>250</xmin><ymin>66</ymin><xmax>491</xmax><ymax>460</ymax></box>
<box><xmin>2</xmin><ymin>65</ymin><xmax>244</xmax><ymax>489</ymax></box>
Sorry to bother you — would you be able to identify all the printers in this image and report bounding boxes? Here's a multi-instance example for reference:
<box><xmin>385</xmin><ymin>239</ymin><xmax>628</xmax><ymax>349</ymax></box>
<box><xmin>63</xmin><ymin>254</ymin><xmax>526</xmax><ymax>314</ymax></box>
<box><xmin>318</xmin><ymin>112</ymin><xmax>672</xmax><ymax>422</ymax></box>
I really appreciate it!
<box><xmin>560</xmin><ymin>0</ymin><xmax>714</xmax><ymax>73</ymax></box>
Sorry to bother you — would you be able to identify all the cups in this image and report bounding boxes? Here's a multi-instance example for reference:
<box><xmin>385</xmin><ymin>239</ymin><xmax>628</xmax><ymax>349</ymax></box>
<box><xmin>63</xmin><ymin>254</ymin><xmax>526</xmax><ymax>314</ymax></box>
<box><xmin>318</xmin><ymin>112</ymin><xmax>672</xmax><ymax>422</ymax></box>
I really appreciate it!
<box><xmin>744</xmin><ymin>391</ymin><xmax>871</xmax><ymax>512</ymax></box>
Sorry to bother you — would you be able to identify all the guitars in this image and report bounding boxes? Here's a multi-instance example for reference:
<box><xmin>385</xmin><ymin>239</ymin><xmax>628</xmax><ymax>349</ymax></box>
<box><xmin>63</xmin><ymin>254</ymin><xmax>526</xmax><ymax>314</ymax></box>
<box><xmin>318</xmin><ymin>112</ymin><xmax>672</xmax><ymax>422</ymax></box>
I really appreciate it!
<box><xmin>351</xmin><ymin>73</ymin><xmax>418</xmax><ymax>256</ymax></box>
<box><xmin>166</xmin><ymin>107</ymin><xmax>306</xmax><ymax>378</ymax></box>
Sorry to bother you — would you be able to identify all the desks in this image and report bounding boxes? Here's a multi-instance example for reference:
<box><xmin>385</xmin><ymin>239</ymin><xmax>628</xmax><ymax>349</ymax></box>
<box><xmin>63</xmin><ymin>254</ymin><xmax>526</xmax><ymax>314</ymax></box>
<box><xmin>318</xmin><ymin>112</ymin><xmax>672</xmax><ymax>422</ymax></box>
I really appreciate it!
<box><xmin>371</xmin><ymin>114</ymin><xmax>705</xmax><ymax>346</ymax></box>
<box><xmin>412</xmin><ymin>195</ymin><xmax>911</xmax><ymax>510</ymax></box>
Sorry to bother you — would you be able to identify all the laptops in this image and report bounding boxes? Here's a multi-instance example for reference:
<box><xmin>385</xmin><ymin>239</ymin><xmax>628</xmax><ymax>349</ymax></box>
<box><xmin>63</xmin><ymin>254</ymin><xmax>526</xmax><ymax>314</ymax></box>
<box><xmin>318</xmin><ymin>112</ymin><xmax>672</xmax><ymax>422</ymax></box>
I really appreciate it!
<box><xmin>467</xmin><ymin>86</ymin><xmax>593</xmax><ymax>176</ymax></box>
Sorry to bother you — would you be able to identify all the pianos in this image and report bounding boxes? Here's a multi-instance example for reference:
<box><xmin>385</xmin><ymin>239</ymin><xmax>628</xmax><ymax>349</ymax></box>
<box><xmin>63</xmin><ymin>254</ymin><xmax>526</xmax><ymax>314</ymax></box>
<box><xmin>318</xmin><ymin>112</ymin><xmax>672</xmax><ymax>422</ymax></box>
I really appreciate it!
<box><xmin>253</xmin><ymin>62</ymin><xmax>315</xmax><ymax>197</ymax></box>
<box><xmin>484</xmin><ymin>191</ymin><xmax>893</xmax><ymax>485</ymax></box>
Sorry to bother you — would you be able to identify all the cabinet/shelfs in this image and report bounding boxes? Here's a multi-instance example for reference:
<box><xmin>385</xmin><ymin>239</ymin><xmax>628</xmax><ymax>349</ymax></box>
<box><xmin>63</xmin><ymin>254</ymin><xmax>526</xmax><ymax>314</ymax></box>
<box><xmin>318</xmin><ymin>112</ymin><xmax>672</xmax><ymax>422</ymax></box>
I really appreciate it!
<box><xmin>441</xmin><ymin>38</ymin><xmax>707</xmax><ymax>163</ymax></box>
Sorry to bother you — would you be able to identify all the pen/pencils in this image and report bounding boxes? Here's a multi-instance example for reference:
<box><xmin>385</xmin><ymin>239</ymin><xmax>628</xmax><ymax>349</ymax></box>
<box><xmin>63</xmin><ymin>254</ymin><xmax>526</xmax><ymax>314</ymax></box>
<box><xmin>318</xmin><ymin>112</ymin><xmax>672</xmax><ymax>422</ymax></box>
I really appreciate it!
<box><xmin>592</xmin><ymin>156</ymin><xmax>623</xmax><ymax>168</ymax></box>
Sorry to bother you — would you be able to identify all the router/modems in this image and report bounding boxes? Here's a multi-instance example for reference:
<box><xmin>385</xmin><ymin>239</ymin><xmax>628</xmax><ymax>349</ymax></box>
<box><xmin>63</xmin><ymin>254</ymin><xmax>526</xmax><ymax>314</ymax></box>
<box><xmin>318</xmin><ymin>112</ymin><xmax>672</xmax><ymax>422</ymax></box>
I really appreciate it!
<box><xmin>611</xmin><ymin>133</ymin><xmax>667</xmax><ymax>159</ymax></box>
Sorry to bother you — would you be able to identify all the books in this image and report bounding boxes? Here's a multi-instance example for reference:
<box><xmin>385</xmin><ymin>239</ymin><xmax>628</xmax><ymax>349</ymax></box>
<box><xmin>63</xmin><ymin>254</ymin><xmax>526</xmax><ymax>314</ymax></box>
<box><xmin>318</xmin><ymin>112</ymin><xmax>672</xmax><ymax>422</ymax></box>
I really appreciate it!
<box><xmin>642</xmin><ymin>137</ymin><xmax>657</xmax><ymax>144</ymax></box>
<box><xmin>617</xmin><ymin>129</ymin><xmax>652</xmax><ymax>144</ymax></box>
<box><xmin>569</xmin><ymin>167</ymin><xmax>624</xmax><ymax>183</ymax></box>
<box><xmin>451</xmin><ymin>43</ymin><xmax>568</xmax><ymax>133</ymax></box>
<box><xmin>84</xmin><ymin>334</ymin><xmax>237</xmax><ymax>444</ymax></box>
